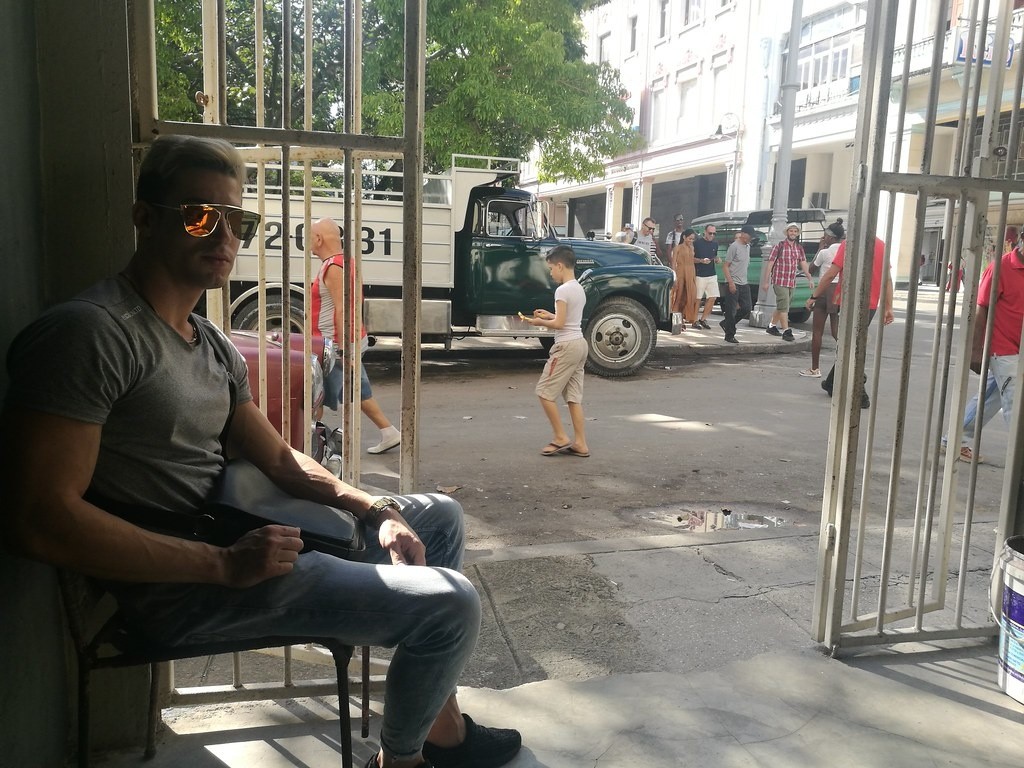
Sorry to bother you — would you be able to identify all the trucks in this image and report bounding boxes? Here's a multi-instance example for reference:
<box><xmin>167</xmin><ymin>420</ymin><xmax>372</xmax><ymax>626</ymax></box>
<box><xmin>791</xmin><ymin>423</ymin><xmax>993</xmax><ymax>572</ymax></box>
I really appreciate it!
<box><xmin>231</xmin><ymin>153</ymin><xmax>681</xmax><ymax>378</ymax></box>
<box><xmin>689</xmin><ymin>208</ymin><xmax>827</xmax><ymax>322</ymax></box>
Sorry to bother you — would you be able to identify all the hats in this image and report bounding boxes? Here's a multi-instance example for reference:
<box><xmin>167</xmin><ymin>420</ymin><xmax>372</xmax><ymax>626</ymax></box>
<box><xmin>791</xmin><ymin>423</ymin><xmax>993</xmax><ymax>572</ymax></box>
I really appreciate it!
<box><xmin>786</xmin><ymin>223</ymin><xmax>800</xmax><ymax>231</ymax></box>
<box><xmin>625</xmin><ymin>223</ymin><xmax>630</xmax><ymax>228</ymax></box>
<box><xmin>741</xmin><ymin>226</ymin><xmax>755</xmax><ymax>235</ymax></box>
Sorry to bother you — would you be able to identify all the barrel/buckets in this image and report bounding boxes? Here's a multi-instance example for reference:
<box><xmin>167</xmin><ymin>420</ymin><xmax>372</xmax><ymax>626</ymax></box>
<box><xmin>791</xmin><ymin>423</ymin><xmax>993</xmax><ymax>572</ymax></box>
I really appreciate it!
<box><xmin>988</xmin><ymin>534</ymin><xmax>1024</xmax><ymax>705</ymax></box>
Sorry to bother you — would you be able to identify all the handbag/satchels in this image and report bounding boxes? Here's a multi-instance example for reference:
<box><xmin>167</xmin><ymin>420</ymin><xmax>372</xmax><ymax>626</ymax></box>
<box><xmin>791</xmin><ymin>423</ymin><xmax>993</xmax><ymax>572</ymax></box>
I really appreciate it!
<box><xmin>195</xmin><ymin>458</ymin><xmax>366</xmax><ymax>560</ymax></box>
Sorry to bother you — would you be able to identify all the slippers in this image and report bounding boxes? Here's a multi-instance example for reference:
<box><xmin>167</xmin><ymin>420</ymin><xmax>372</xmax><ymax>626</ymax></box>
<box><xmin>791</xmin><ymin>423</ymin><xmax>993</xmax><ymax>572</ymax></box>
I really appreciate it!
<box><xmin>560</xmin><ymin>447</ymin><xmax>590</xmax><ymax>457</ymax></box>
<box><xmin>542</xmin><ymin>441</ymin><xmax>573</xmax><ymax>455</ymax></box>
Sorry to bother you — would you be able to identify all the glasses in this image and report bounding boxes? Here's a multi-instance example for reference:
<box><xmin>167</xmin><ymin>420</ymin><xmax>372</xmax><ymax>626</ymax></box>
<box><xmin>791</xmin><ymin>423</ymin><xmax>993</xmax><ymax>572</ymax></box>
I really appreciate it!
<box><xmin>676</xmin><ymin>218</ymin><xmax>683</xmax><ymax>222</ymax></box>
<box><xmin>143</xmin><ymin>203</ymin><xmax>261</xmax><ymax>241</ymax></box>
<box><xmin>644</xmin><ymin>224</ymin><xmax>655</xmax><ymax>230</ymax></box>
<box><xmin>709</xmin><ymin>232</ymin><xmax>716</xmax><ymax>235</ymax></box>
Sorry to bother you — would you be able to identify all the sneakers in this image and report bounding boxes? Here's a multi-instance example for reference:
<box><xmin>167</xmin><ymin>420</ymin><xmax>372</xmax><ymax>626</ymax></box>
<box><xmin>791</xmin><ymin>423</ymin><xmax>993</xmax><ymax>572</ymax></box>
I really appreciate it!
<box><xmin>422</xmin><ymin>713</ymin><xmax>521</xmax><ymax>768</ymax></box>
<box><xmin>767</xmin><ymin>326</ymin><xmax>782</xmax><ymax>336</ymax></box>
<box><xmin>364</xmin><ymin>752</ymin><xmax>441</xmax><ymax>768</ymax></box>
<box><xmin>783</xmin><ymin>329</ymin><xmax>795</xmax><ymax>341</ymax></box>
<box><xmin>798</xmin><ymin>368</ymin><xmax>822</xmax><ymax>378</ymax></box>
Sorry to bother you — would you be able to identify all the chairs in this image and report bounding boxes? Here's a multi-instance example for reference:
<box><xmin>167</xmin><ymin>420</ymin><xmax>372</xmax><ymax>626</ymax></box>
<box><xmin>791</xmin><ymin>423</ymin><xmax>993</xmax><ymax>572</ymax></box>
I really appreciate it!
<box><xmin>58</xmin><ymin>564</ymin><xmax>369</xmax><ymax>767</ymax></box>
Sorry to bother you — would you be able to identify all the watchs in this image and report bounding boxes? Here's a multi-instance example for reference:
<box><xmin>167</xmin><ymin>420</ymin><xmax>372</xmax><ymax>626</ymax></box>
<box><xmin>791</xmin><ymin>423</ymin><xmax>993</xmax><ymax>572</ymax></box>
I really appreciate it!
<box><xmin>335</xmin><ymin>349</ymin><xmax>343</xmax><ymax>356</ymax></box>
<box><xmin>366</xmin><ymin>497</ymin><xmax>402</xmax><ymax>524</ymax></box>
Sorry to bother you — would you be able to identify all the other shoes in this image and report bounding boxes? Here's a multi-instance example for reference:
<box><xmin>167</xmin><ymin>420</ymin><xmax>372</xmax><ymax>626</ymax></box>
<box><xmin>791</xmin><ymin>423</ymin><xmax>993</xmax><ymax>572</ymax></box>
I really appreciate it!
<box><xmin>719</xmin><ymin>320</ymin><xmax>726</xmax><ymax>332</ymax></box>
<box><xmin>699</xmin><ymin>319</ymin><xmax>711</xmax><ymax>329</ymax></box>
<box><xmin>940</xmin><ymin>445</ymin><xmax>984</xmax><ymax>464</ymax></box>
<box><xmin>822</xmin><ymin>380</ymin><xmax>832</xmax><ymax>396</ymax></box>
<box><xmin>367</xmin><ymin>433</ymin><xmax>400</xmax><ymax>454</ymax></box>
<box><xmin>725</xmin><ymin>333</ymin><xmax>739</xmax><ymax>343</ymax></box>
<box><xmin>682</xmin><ymin>325</ymin><xmax>686</xmax><ymax>331</ymax></box>
<box><xmin>692</xmin><ymin>321</ymin><xmax>702</xmax><ymax>329</ymax></box>
<box><xmin>861</xmin><ymin>395</ymin><xmax>870</xmax><ymax>408</ymax></box>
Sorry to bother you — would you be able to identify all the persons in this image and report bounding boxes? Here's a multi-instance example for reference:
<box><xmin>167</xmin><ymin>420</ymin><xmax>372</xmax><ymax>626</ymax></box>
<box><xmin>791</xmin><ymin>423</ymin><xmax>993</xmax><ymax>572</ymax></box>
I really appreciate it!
<box><xmin>305</xmin><ymin>216</ymin><xmax>401</xmax><ymax>456</ymax></box>
<box><xmin>798</xmin><ymin>219</ymin><xmax>846</xmax><ymax>378</ymax></box>
<box><xmin>670</xmin><ymin>228</ymin><xmax>699</xmax><ymax>323</ymax></box>
<box><xmin>762</xmin><ymin>222</ymin><xmax>814</xmax><ymax>340</ymax></box>
<box><xmin>615</xmin><ymin>214</ymin><xmax>686</xmax><ymax>319</ymax></box>
<box><xmin>807</xmin><ymin>222</ymin><xmax>894</xmax><ymax>409</ymax></box>
<box><xmin>517</xmin><ymin>246</ymin><xmax>591</xmax><ymax>457</ymax></box>
<box><xmin>940</xmin><ymin>222</ymin><xmax>1023</xmax><ymax>464</ymax></box>
<box><xmin>693</xmin><ymin>224</ymin><xmax>723</xmax><ymax>328</ymax></box>
<box><xmin>918</xmin><ymin>252</ymin><xmax>966</xmax><ymax>292</ymax></box>
<box><xmin>718</xmin><ymin>226</ymin><xmax>752</xmax><ymax>343</ymax></box>
<box><xmin>4</xmin><ymin>133</ymin><xmax>521</xmax><ymax>766</ymax></box>
